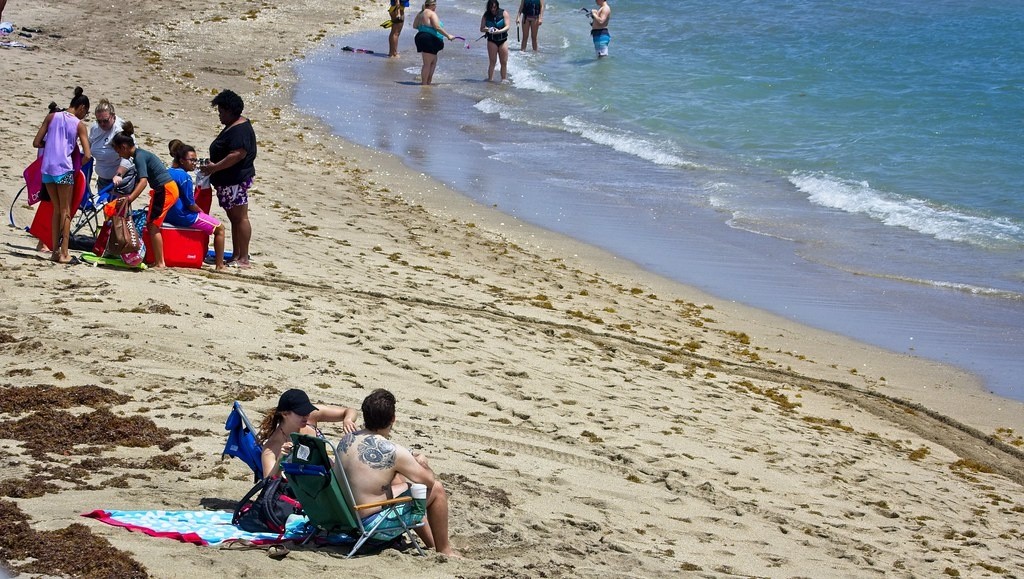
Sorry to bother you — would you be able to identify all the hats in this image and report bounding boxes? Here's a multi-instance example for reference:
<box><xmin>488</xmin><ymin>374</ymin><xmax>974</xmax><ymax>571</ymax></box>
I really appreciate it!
<box><xmin>276</xmin><ymin>389</ymin><xmax>319</xmax><ymax>416</ymax></box>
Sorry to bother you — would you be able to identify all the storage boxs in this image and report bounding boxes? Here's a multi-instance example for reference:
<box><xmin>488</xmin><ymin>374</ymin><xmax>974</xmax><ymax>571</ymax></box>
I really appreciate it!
<box><xmin>142</xmin><ymin>221</ymin><xmax>210</xmax><ymax>268</ymax></box>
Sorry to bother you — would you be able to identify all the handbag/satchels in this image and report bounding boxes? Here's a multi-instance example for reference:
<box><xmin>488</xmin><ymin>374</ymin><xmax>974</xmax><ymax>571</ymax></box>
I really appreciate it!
<box><xmin>194</xmin><ymin>170</ymin><xmax>212</xmax><ymax>215</ymax></box>
<box><xmin>93</xmin><ymin>199</ymin><xmax>150</xmax><ymax>267</ymax></box>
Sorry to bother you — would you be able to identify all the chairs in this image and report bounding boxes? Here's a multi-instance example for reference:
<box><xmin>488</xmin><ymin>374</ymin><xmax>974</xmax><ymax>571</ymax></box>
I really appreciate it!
<box><xmin>72</xmin><ymin>152</ymin><xmax>135</xmax><ymax>239</ymax></box>
<box><xmin>231</xmin><ymin>402</ymin><xmax>304</xmax><ymax>533</ymax></box>
<box><xmin>280</xmin><ymin>433</ymin><xmax>429</xmax><ymax>558</ymax></box>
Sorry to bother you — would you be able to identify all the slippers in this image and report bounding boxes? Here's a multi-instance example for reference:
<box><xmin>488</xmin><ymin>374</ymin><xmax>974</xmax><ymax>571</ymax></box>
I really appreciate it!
<box><xmin>226</xmin><ymin>261</ymin><xmax>251</xmax><ymax>269</ymax></box>
<box><xmin>67</xmin><ymin>255</ymin><xmax>82</xmax><ymax>264</ymax></box>
<box><xmin>268</xmin><ymin>544</ymin><xmax>290</xmax><ymax>557</ymax></box>
<box><xmin>220</xmin><ymin>538</ymin><xmax>268</xmax><ymax>550</ymax></box>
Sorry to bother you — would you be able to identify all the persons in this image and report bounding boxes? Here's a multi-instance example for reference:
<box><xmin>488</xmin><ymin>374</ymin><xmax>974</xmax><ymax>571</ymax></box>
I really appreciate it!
<box><xmin>255</xmin><ymin>389</ymin><xmax>356</xmax><ymax>486</ymax></box>
<box><xmin>333</xmin><ymin>389</ymin><xmax>454</xmax><ymax>556</ymax></box>
<box><xmin>33</xmin><ymin>86</ymin><xmax>257</xmax><ymax>269</ymax></box>
<box><xmin>590</xmin><ymin>0</ymin><xmax>611</xmax><ymax>58</ymax></box>
<box><xmin>413</xmin><ymin>0</ymin><xmax>455</xmax><ymax>85</ymax></box>
<box><xmin>480</xmin><ymin>0</ymin><xmax>510</xmax><ymax>81</ymax></box>
<box><xmin>388</xmin><ymin>0</ymin><xmax>409</xmax><ymax>57</ymax></box>
<box><xmin>515</xmin><ymin>0</ymin><xmax>544</xmax><ymax>51</ymax></box>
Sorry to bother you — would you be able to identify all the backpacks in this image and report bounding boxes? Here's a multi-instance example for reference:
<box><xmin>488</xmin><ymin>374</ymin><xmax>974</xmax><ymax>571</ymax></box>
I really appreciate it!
<box><xmin>232</xmin><ymin>476</ymin><xmax>303</xmax><ymax>533</ymax></box>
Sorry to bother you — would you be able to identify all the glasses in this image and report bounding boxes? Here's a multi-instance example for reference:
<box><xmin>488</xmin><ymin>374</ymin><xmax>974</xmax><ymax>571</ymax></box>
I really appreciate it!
<box><xmin>95</xmin><ymin>115</ymin><xmax>112</xmax><ymax>124</ymax></box>
<box><xmin>183</xmin><ymin>158</ymin><xmax>197</xmax><ymax>162</ymax></box>
<box><xmin>85</xmin><ymin>107</ymin><xmax>90</xmax><ymax>117</ymax></box>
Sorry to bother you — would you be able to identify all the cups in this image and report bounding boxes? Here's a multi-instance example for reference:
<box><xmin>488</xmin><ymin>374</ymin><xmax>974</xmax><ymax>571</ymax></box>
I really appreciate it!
<box><xmin>410</xmin><ymin>483</ymin><xmax>427</xmax><ymax>499</ymax></box>
